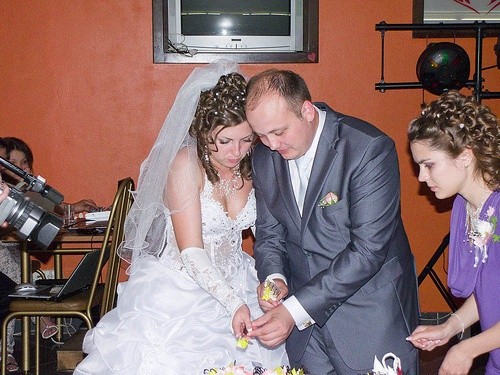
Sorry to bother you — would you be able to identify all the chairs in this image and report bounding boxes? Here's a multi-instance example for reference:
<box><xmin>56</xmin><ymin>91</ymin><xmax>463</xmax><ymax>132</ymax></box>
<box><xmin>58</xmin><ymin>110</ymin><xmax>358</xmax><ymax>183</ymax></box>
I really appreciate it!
<box><xmin>0</xmin><ymin>177</ymin><xmax>138</xmax><ymax>375</ymax></box>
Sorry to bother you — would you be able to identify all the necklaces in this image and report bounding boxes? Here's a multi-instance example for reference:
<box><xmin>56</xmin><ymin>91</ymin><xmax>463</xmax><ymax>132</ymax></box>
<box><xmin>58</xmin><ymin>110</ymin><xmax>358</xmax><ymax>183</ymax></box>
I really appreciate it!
<box><xmin>464</xmin><ymin>203</ymin><xmax>483</xmax><ymax>242</ymax></box>
<box><xmin>205</xmin><ymin>155</ymin><xmax>241</xmax><ymax>199</ymax></box>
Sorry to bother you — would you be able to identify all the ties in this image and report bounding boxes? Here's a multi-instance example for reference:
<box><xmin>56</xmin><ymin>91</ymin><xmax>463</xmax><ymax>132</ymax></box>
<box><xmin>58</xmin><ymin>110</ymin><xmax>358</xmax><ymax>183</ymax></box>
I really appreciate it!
<box><xmin>294</xmin><ymin>153</ymin><xmax>312</xmax><ymax>217</ymax></box>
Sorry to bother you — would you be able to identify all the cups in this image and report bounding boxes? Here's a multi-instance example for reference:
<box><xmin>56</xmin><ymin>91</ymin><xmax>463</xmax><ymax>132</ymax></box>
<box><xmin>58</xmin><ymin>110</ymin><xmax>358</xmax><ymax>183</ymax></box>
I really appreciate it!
<box><xmin>63</xmin><ymin>203</ymin><xmax>75</xmax><ymax>228</ymax></box>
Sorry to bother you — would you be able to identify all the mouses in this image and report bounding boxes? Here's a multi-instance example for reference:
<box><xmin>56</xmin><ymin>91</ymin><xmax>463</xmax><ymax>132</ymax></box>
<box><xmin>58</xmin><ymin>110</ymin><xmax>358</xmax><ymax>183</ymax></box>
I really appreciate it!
<box><xmin>15</xmin><ymin>283</ymin><xmax>39</xmax><ymax>291</ymax></box>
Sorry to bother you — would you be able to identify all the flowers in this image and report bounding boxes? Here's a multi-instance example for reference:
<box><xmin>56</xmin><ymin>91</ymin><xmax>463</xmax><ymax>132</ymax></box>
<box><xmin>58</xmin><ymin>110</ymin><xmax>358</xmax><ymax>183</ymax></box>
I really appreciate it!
<box><xmin>318</xmin><ymin>191</ymin><xmax>337</xmax><ymax>209</ymax></box>
<box><xmin>469</xmin><ymin>206</ymin><xmax>500</xmax><ymax>266</ymax></box>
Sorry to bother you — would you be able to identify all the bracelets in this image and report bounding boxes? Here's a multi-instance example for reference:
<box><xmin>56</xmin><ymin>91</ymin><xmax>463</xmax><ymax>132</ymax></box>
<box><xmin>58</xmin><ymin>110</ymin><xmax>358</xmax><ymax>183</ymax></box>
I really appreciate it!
<box><xmin>451</xmin><ymin>314</ymin><xmax>465</xmax><ymax>339</ymax></box>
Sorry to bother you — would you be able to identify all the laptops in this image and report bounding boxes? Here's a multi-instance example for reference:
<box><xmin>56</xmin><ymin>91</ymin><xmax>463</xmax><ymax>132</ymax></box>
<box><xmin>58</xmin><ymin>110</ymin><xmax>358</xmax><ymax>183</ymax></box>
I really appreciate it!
<box><xmin>8</xmin><ymin>246</ymin><xmax>111</xmax><ymax>301</ymax></box>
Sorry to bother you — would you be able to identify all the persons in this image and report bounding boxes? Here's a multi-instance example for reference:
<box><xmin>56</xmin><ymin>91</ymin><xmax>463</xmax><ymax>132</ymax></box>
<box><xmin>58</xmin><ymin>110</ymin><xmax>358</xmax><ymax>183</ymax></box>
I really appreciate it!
<box><xmin>73</xmin><ymin>60</ymin><xmax>291</xmax><ymax>375</ymax></box>
<box><xmin>242</xmin><ymin>69</ymin><xmax>419</xmax><ymax>375</ymax></box>
<box><xmin>0</xmin><ymin>137</ymin><xmax>97</xmax><ymax>372</ymax></box>
<box><xmin>406</xmin><ymin>91</ymin><xmax>500</xmax><ymax>375</ymax></box>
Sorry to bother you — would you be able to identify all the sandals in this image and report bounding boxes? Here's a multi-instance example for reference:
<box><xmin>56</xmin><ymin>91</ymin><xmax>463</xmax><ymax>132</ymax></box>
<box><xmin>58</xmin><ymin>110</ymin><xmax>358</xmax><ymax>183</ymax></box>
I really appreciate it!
<box><xmin>37</xmin><ymin>316</ymin><xmax>59</xmax><ymax>339</ymax></box>
<box><xmin>6</xmin><ymin>353</ymin><xmax>19</xmax><ymax>372</ymax></box>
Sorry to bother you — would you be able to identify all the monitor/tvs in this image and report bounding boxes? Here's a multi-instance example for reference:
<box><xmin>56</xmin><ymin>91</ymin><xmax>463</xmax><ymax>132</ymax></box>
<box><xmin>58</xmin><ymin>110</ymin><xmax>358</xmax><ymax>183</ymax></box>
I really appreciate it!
<box><xmin>166</xmin><ymin>0</ymin><xmax>303</xmax><ymax>55</ymax></box>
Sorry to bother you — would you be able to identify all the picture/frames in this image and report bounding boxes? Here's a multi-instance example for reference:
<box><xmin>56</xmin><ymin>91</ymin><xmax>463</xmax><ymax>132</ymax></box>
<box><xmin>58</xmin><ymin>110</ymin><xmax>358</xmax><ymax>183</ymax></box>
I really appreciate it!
<box><xmin>412</xmin><ymin>0</ymin><xmax>500</xmax><ymax>39</ymax></box>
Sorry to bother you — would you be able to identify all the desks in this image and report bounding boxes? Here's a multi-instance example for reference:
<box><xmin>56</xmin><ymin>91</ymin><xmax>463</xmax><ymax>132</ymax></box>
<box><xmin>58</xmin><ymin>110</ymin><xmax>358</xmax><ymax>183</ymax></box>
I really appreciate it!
<box><xmin>0</xmin><ymin>221</ymin><xmax>132</xmax><ymax>375</ymax></box>
